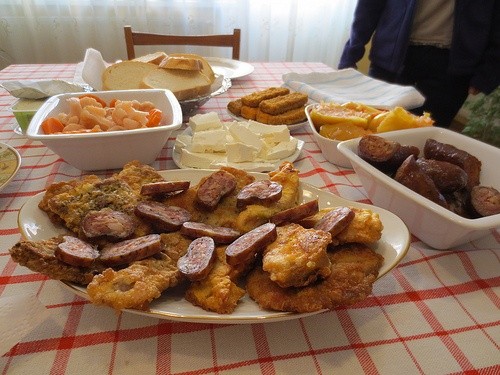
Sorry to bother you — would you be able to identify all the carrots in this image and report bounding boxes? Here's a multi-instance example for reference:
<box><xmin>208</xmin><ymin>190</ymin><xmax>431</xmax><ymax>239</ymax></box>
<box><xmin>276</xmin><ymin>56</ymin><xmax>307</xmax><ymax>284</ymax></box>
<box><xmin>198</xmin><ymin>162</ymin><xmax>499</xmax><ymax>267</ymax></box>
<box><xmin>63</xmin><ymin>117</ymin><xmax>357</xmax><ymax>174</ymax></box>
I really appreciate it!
<box><xmin>110</xmin><ymin>99</ymin><xmax>116</xmax><ymax>108</ymax></box>
<box><xmin>78</xmin><ymin>94</ymin><xmax>106</xmax><ymax>108</ymax></box>
<box><xmin>144</xmin><ymin>109</ymin><xmax>162</xmax><ymax>127</ymax></box>
<box><xmin>42</xmin><ymin>117</ymin><xmax>64</xmax><ymax>134</ymax></box>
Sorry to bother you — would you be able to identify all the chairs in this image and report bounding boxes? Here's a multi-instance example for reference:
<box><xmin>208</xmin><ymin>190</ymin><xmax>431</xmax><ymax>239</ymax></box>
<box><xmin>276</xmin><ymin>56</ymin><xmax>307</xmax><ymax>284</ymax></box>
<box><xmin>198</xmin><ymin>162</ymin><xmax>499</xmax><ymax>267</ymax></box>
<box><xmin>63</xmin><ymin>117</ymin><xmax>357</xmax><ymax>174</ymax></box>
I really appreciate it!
<box><xmin>123</xmin><ymin>26</ymin><xmax>243</xmax><ymax>62</ymax></box>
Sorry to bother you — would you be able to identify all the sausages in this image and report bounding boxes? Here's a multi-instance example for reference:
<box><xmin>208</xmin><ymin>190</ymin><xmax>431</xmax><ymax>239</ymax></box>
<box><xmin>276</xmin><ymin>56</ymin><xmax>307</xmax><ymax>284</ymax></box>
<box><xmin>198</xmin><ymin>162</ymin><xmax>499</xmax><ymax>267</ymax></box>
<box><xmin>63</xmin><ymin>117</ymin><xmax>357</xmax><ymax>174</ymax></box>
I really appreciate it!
<box><xmin>358</xmin><ymin>134</ymin><xmax>500</xmax><ymax>220</ymax></box>
<box><xmin>53</xmin><ymin>169</ymin><xmax>355</xmax><ymax>283</ymax></box>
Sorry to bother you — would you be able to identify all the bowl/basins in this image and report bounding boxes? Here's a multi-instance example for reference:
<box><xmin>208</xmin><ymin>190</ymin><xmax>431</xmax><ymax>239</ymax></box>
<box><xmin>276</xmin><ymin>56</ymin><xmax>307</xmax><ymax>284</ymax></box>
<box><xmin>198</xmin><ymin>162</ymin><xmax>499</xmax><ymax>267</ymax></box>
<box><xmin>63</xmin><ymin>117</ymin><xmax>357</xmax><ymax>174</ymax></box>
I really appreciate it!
<box><xmin>305</xmin><ymin>102</ymin><xmax>388</xmax><ymax>168</ymax></box>
<box><xmin>27</xmin><ymin>88</ymin><xmax>183</xmax><ymax>172</ymax></box>
<box><xmin>10</xmin><ymin>98</ymin><xmax>47</xmax><ymax>133</ymax></box>
<box><xmin>336</xmin><ymin>127</ymin><xmax>500</xmax><ymax>250</ymax></box>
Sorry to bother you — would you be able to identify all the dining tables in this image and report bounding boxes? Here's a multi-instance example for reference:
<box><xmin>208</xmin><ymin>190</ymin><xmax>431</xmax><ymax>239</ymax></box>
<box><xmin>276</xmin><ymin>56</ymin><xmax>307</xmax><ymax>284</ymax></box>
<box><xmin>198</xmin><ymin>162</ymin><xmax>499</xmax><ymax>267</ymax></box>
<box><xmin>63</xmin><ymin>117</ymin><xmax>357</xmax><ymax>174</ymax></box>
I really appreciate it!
<box><xmin>0</xmin><ymin>61</ymin><xmax>500</xmax><ymax>375</ymax></box>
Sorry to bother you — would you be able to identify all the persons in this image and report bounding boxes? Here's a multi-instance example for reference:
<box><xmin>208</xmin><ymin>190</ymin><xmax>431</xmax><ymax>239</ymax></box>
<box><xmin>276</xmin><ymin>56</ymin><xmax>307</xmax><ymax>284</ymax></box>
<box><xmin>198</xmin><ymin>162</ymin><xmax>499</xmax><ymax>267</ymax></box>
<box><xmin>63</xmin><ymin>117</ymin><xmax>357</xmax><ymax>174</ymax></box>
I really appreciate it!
<box><xmin>338</xmin><ymin>0</ymin><xmax>500</xmax><ymax>130</ymax></box>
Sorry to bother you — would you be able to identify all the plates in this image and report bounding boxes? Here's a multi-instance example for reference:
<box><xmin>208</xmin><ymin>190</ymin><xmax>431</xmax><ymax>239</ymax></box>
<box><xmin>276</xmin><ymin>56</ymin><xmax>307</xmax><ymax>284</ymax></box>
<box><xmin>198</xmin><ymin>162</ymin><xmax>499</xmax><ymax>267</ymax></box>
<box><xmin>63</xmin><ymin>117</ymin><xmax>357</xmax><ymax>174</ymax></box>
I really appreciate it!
<box><xmin>173</xmin><ymin>122</ymin><xmax>305</xmax><ymax>172</ymax></box>
<box><xmin>203</xmin><ymin>57</ymin><xmax>255</xmax><ymax>79</ymax></box>
<box><xmin>177</xmin><ymin>74</ymin><xmax>232</xmax><ymax>114</ymax></box>
<box><xmin>226</xmin><ymin>92</ymin><xmax>309</xmax><ymax>132</ymax></box>
<box><xmin>13</xmin><ymin>124</ymin><xmax>32</xmax><ymax>137</ymax></box>
<box><xmin>0</xmin><ymin>140</ymin><xmax>21</xmax><ymax>194</ymax></box>
<box><xmin>16</xmin><ymin>168</ymin><xmax>411</xmax><ymax>324</ymax></box>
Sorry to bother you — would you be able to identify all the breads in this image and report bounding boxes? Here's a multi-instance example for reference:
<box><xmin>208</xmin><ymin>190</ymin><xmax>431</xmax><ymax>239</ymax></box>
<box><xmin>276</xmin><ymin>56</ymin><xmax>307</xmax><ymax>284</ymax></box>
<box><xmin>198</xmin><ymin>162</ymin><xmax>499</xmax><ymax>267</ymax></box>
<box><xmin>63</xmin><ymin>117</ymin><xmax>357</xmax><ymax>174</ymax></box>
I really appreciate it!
<box><xmin>102</xmin><ymin>51</ymin><xmax>214</xmax><ymax>100</ymax></box>
<box><xmin>227</xmin><ymin>86</ymin><xmax>310</xmax><ymax>125</ymax></box>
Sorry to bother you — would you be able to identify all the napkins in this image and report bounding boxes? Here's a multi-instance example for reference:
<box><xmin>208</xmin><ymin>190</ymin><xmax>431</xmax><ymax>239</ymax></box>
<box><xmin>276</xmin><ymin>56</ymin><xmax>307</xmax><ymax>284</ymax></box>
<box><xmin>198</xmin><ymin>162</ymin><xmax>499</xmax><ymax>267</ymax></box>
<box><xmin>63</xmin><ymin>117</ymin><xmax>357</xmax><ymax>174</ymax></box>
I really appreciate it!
<box><xmin>282</xmin><ymin>66</ymin><xmax>426</xmax><ymax>109</ymax></box>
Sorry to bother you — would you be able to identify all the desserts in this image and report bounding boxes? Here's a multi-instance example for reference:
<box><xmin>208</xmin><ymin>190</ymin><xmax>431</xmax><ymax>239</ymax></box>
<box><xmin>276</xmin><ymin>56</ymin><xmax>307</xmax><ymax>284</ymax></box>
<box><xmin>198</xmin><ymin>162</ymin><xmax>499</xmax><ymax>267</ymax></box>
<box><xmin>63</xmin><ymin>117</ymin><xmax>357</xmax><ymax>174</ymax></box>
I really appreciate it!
<box><xmin>174</xmin><ymin>111</ymin><xmax>298</xmax><ymax>172</ymax></box>
